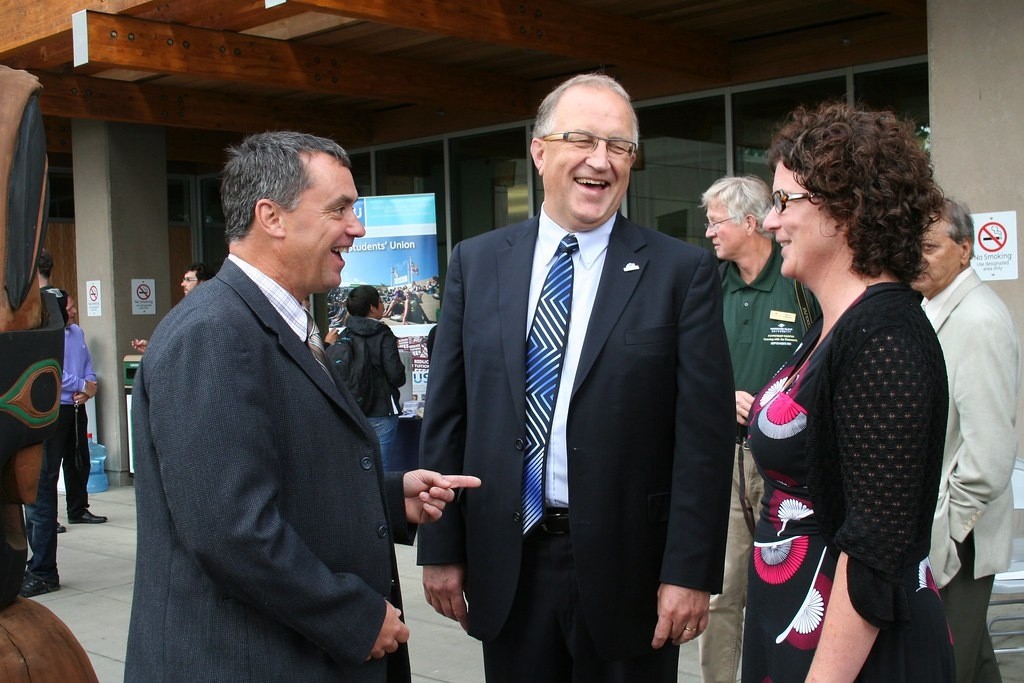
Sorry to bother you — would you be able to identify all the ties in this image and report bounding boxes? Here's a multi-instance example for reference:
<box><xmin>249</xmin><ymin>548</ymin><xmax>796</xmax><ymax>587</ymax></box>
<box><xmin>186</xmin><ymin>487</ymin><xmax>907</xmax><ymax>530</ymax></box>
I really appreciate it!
<box><xmin>522</xmin><ymin>234</ymin><xmax>579</xmax><ymax>536</ymax></box>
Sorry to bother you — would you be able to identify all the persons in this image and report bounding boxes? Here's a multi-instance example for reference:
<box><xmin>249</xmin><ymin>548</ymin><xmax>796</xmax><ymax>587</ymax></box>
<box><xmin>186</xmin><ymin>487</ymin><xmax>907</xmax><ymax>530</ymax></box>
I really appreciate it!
<box><xmin>125</xmin><ymin>131</ymin><xmax>481</xmax><ymax>683</ymax></box>
<box><xmin>416</xmin><ymin>75</ymin><xmax>738</xmax><ymax>683</ymax></box>
<box><xmin>131</xmin><ymin>264</ymin><xmax>213</xmax><ymax>354</ymax></box>
<box><xmin>328</xmin><ymin>276</ymin><xmax>440</xmax><ymax>328</ymax></box>
<box><xmin>19</xmin><ymin>251</ymin><xmax>70</xmax><ymax>597</ymax></box>
<box><xmin>742</xmin><ymin>105</ymin><xmax>955</xmax><ymax>683</ymax></box>
<box><xmin>56</xmin><ymin>292</ymin><xmax>107</xmax><ymax>533</ymax></box>
<box><xmin>301</xmin><ymin>284</ymin><xmax>408</xmax><ymax>472</ymax></box>
<box><xmin>699</xmin><ymin>176</ymin><xmax>816</xmax><ymax>683</ymax></box>
<box><xmin>911</xmin><ymin>198</ymin><xmax>1022</xmax><ymax>683</ymax></box>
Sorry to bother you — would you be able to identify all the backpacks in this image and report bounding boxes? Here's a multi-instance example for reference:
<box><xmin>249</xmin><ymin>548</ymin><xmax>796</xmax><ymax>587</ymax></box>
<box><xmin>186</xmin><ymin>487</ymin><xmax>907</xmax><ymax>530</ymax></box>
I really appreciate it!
<box><xmin>325</xmin><ymin>326</ymin><xmax>378</xmax><ymax>416</ymax></box>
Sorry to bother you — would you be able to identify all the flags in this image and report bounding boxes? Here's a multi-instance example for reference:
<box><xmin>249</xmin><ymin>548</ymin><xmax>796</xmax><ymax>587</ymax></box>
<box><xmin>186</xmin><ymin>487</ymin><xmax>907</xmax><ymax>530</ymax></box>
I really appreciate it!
<box><xmin>392</xmin><ymin>267</ymin><xmax>399</xmax><ymax>277</ymax></box>
<box><xmin>408</xmin><ymin>260</ymin><xmax>419</xmax><ymax>274</ymax></box>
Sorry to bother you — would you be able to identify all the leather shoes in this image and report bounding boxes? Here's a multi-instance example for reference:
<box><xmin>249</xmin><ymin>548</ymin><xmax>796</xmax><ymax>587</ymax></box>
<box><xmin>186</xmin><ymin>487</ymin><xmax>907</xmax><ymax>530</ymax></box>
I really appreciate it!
<box><xmin>69</xmin><ymin>511</ymin><xmax>108</xmax><ymax>522</ymax></box>
<box><xmin>56</xmin><ymin>520</ymin><xmax>65</xmax><ymax>533</ymax></box>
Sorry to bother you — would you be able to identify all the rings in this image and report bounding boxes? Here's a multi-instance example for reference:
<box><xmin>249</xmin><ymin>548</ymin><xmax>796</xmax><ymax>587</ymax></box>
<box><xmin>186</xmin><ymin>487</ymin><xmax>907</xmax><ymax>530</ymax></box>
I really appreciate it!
<box><xmin>685</xmin><ymin>628</ymin><xmax>696</xmax><ymax>631</ymax></box>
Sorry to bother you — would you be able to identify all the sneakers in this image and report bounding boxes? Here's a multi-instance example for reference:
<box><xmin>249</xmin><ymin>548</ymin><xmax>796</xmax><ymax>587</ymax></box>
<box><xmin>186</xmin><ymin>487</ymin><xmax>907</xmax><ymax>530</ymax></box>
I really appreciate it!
<box><xmin>20</xmin><ymin>564</ymin><xmax>60</xmax><ymax>596</ymax></box>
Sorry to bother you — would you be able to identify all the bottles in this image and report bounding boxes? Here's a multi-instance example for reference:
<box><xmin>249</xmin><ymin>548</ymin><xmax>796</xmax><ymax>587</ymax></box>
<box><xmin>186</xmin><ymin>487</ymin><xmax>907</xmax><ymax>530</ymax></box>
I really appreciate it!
<box><xmin>87</xmin><ymin>434</ymin><xmax>109</xmax><ymax>494</ymax></box>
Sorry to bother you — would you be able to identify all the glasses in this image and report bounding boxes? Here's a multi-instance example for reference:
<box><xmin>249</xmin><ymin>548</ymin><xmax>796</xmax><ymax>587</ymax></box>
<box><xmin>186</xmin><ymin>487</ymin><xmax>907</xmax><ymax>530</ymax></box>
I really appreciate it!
<box><xmin>541</xmin><ymin>133</ymin><xmax>636</xmax><ymax>162</ymax></box>
<box><xmin>704</xmin><ymin>215</ymin><xmax>737</xmax><ymax>232</ymax></box>
<box><xmin>771</xmin><ymin>187</ymin><xmax>816</xmax><ymax>215</ymax></box>
